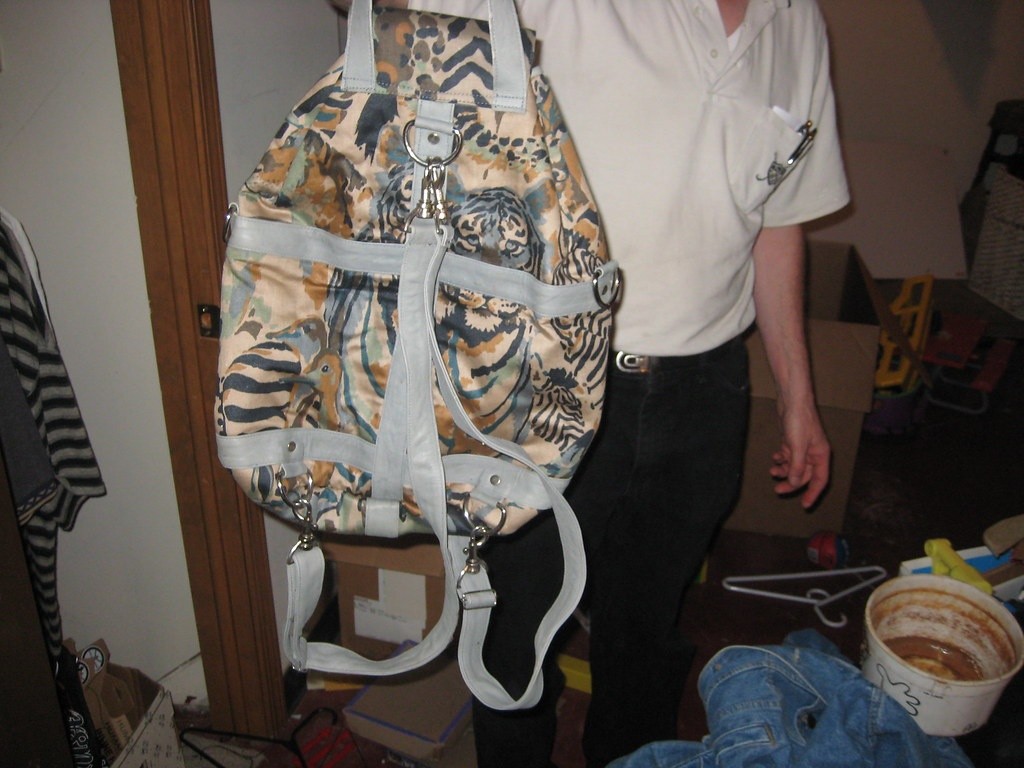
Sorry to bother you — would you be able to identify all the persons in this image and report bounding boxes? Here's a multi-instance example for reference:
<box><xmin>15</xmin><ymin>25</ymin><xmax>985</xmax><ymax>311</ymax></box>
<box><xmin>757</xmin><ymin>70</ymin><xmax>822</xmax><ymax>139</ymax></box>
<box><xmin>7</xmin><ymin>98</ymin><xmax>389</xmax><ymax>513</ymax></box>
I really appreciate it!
<box><xmin>333</xmin><ymin>1</ymin><xmax>855</xmax><ymax>768</ymax></box>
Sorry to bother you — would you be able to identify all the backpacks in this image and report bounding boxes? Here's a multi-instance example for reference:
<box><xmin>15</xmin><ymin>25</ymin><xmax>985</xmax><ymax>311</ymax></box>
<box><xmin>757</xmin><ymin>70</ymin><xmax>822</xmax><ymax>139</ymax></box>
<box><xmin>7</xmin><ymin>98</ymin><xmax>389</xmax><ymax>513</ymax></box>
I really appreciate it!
<box><xmin>215</xmin><ymin>0</ymin><xmax>619</xmax><ymax>710</ymax></box>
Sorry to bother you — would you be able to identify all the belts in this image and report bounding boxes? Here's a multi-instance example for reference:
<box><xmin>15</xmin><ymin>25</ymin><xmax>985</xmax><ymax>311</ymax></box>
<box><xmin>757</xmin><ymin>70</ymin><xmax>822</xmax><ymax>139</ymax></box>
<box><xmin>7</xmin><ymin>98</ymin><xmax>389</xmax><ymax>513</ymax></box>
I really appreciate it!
<box><xmin>615</xmin><ymin>329</ymin><xmax>744</xmax><ymax>374</ymax></box>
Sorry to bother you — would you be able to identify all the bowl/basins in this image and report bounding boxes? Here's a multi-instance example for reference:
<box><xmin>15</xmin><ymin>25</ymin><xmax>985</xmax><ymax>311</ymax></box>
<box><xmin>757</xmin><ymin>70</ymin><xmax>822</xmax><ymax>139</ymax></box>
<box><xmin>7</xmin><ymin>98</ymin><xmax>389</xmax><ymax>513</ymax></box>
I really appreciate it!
<box><xmin>863</xmin><ymin>574</ymin><xmax>1024</xmax><ymax>737</ymax></box>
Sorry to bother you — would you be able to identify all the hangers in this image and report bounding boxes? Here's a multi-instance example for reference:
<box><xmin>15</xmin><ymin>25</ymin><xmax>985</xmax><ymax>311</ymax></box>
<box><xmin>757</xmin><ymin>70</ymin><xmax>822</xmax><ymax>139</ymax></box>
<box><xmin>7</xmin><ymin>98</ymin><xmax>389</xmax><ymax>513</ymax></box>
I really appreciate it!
<box><xmin>721</xmin><ymin>565</ymin><xmax>887</xmax><ymax>628</ymax></box>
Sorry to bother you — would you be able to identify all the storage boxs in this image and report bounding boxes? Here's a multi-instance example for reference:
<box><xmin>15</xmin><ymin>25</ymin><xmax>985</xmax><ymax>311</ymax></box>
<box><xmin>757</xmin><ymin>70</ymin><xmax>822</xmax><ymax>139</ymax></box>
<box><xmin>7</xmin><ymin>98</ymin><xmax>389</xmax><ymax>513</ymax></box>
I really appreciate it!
<box><xmin>64</xmin><ymin>636</ymin><xmax>185</xmax><ymax>768</ymax></box>
<box><xmin>342</xmin><ymin>640</ymin><xmax>473</xmax><ymax>762</ymax></box>
<box><xmin>324</xmin><ymin>541</ymin><xmax>445</xmax><ymax>662</ymax></box>
<box><xmin>723</xmin><ymin>238</ymin><xmax>926</xmax><ymax>538</ymax></box>
